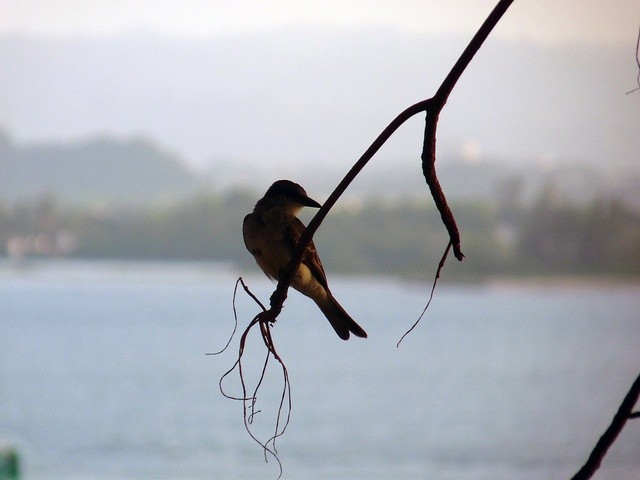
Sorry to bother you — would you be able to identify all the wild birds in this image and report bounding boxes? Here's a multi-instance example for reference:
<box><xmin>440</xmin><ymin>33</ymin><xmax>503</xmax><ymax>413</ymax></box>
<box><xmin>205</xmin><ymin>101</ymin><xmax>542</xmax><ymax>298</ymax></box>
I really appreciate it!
<box><xmin>242</xmin><ymin>179</ymin><xmax>370</xmax><ymax>341</ymax></box>
